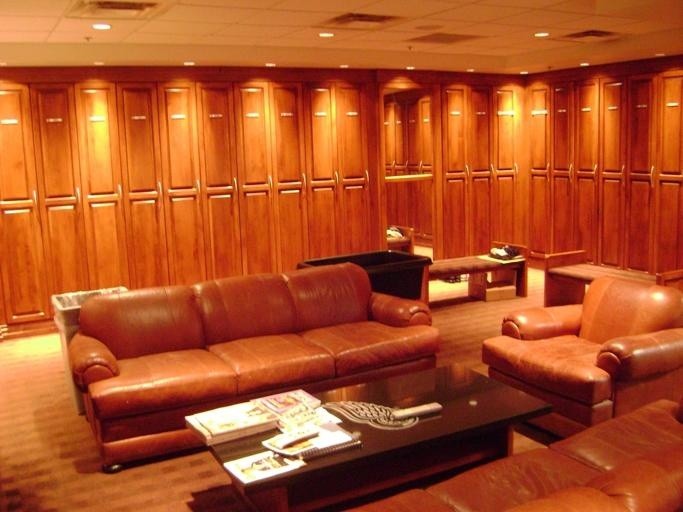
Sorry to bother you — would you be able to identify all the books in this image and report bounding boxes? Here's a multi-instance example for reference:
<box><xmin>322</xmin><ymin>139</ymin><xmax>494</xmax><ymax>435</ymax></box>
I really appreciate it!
<box><xmin>184</xmin><ymin>388</ymin><xmax>364</xmax><ymax>486</ymax></box>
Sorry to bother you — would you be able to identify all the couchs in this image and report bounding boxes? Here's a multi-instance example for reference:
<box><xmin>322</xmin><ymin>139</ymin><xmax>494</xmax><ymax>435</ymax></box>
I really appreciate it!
<box><xmin>348</xmin><ymin>399</ymin><xmax>682</xmax><ymax>511</ymax></box>
<box><xmin>483</xmin><ymin>275</ymin><xmax>682</xmax><ymax>447</ymax></box>
<box><xmin>70</xmin><ymin>261</ymin><xmax>439</xmax><ymax>472</ymax></box>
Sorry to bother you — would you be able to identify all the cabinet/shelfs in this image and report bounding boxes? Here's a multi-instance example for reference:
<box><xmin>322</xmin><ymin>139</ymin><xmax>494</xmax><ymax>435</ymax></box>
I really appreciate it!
<box><xmin>384</xmin><ymin>93</ymin><xmax>434</xmax><ymax>244</ymax></box>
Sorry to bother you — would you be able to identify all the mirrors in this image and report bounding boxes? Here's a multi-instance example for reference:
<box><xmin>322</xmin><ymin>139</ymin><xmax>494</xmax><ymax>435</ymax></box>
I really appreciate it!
<box><xmin>384</xmin><ymin>84</ymin><xmax>437</xmax><ymax>261</ymax></box>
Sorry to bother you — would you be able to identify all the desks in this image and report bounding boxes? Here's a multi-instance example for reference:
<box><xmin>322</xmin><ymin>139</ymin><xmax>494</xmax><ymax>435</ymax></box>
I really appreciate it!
<box><xmin>295</xmin><ymin>248</ymin><xmax>432</xmax><ymax>309</ymax></box>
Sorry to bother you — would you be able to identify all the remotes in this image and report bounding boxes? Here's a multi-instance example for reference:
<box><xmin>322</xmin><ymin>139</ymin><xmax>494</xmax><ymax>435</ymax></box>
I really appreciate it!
<box><xmin>268</xmin><ymin>426</ymin><xmax>320</xmax><ymax>449</ymax></box>
<box><xmin>392</xmin><ymin>402</ymin><xmax>443</xmax><ymax>420</ymax></box>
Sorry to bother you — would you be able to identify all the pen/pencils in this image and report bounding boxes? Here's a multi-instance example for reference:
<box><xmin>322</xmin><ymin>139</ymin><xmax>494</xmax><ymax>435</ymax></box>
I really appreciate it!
<box><xmin>283</xmin><ymin>433</ymin><xmax>318</xmax><ymax>448</ymax></box>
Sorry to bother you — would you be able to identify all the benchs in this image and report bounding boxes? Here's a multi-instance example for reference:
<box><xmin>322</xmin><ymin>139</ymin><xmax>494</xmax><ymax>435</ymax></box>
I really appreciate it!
<box><xmin>421</xmin><ymin>255</ymin><xmax>527</xmax><ymax>306</ymax></box>
<box><xmin>542</xmin><ymin>252</ymin><xmax>680</xmax><ymax>314</ymax></box>
<box><xmin>386</xmin><ymin>226</ymin><xmax>416</xmax><ymax>255</ymax></box>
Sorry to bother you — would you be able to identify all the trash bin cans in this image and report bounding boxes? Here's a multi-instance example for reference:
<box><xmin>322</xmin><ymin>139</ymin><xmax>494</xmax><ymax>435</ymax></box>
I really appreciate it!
<box><xmin>51</xmin><ymin>286</ymin><xmax>129</xmax><ymax>416</ymax></box>
